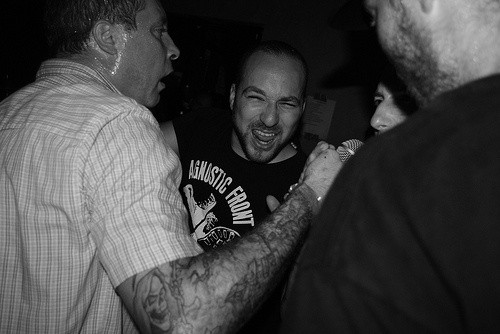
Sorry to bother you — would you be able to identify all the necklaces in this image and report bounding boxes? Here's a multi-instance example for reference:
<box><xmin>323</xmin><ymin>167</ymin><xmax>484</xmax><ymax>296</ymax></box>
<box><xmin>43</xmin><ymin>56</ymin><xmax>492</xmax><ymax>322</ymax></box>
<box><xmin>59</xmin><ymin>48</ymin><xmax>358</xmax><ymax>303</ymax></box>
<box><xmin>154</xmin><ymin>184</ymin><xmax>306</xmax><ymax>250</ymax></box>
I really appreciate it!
<box><xmin>290</xmin><ymin>141</ymin><xmax>299</xmax><ymax>150</ymax></box>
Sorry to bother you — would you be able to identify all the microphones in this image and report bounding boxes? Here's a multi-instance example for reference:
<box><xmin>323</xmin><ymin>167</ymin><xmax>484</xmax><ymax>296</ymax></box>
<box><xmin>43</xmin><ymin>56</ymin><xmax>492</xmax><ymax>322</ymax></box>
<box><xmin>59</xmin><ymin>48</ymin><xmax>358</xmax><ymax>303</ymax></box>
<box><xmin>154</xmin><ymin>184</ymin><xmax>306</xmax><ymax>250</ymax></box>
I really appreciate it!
<box><xmin>335</xmin><ymin>139</ymin><xmax>364</xmax><ymax>162</ymax></box>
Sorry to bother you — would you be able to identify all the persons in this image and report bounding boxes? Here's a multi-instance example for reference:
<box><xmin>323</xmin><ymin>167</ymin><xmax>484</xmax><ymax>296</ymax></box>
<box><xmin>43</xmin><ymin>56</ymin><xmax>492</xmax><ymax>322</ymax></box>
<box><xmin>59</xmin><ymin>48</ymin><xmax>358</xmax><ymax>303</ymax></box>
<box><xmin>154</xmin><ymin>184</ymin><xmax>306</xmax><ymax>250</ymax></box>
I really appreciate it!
<box><xmin>369</xmin><ymin>64</ymin><xmax>426</xmax><ymax>134</ymax></box>
<box><xmin>0</xmin><ymin>0</ymin><xmax>346</xmax><ymax>334</ymax></box>
<box><xmin>275</xmin><ymin>1</ymin><xmax>500</xmax><ymax>334</ymax></box>
<box><xmin>159</xmin><ymin>40</ymin><xmax>309</xmax><ymax>254</ymax></box>
